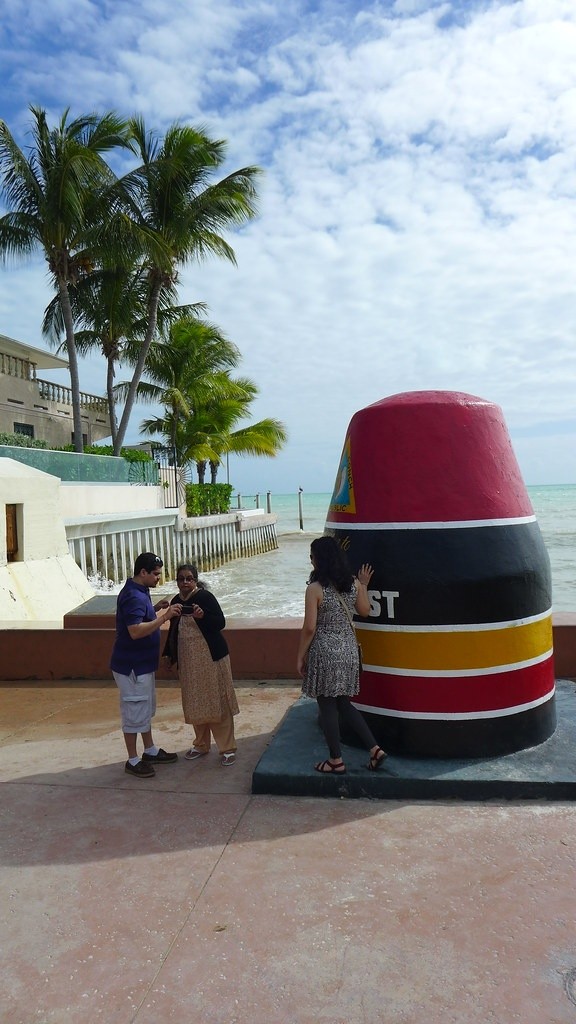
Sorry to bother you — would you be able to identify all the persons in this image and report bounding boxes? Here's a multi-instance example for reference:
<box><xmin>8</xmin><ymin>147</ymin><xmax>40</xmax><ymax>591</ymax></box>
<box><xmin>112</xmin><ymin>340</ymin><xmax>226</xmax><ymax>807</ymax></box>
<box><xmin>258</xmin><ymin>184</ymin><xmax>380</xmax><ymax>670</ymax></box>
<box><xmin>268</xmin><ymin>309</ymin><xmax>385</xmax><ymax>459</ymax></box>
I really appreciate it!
<box><xmin>296</xmin><ymin>536</ymin><xmax>388</xmax><ymax>773</ymax></box>
<box><xmin>109</xmin><ymin>552</ymin><xmax>183</xmax><ymax>778</ymax></box>
<box><xmin>162</xmin><ymin>565</ymin><xmax>240</xmax><ymax>766</ymax></box>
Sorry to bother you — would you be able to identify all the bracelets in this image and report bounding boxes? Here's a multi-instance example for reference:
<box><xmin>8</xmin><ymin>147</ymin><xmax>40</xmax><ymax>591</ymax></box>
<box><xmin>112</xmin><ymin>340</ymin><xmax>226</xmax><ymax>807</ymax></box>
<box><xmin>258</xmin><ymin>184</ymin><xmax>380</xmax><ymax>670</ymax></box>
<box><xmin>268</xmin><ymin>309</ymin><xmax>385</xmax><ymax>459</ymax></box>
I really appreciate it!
<box><xmin>361</xmin><ymin>582</ymin><xmax>368</xmax><ymax>586</ymax></box>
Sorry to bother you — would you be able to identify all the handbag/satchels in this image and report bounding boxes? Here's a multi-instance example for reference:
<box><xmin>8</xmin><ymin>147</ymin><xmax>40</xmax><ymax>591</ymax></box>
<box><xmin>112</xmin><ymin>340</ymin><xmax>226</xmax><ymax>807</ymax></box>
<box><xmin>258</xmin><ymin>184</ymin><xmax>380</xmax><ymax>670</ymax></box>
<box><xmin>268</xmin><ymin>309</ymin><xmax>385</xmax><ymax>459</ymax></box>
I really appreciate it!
<box><xmin>358</xmin><ymin>644</ymin><xmax>364</xmax><ymax>673</ymax></box>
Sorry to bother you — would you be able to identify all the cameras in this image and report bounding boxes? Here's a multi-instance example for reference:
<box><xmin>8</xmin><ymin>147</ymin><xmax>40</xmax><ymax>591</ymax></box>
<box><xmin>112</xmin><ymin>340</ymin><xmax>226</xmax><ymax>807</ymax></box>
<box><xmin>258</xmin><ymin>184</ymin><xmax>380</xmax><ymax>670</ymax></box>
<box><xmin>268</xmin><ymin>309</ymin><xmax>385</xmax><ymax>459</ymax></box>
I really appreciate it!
<box><xmin>182</xmin><ymin>606</ymin><xmax>194</xmax><ymax>614</ymax></box>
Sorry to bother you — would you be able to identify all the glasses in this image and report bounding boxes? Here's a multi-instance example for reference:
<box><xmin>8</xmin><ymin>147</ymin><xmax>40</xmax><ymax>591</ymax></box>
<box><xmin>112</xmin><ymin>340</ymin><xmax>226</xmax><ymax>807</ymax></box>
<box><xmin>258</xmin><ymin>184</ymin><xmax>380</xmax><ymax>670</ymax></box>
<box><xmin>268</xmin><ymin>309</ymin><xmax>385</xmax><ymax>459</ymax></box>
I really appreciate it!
<box><xmin>175</xmin><ymin>577</ymin><xmax>196</xmax><ymax>584</ymax></box>
<box><xmin>310</xmin><ymin>555</ymin><xmax>313</xmax><ymax>560</ymax></box>
<box><xmin>147</xmin><ymin>556</ymin><xmax>161</xmax><ymax>571</ymax></box>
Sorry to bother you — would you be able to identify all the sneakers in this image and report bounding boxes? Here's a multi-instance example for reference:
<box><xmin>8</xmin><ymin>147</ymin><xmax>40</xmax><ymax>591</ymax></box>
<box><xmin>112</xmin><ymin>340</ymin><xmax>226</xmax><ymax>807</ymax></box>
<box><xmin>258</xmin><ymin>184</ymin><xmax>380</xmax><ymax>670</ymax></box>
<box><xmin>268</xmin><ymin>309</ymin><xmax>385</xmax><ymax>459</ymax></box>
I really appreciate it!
<box><xmin>142</xmin><ymin>746</ymin><xmax>178</xmax><ymax>764</ymax></box>
<box><xmin>125</xmin><ymin>758</ymin><xmax>155</xmax><ymax>778</ymax></box>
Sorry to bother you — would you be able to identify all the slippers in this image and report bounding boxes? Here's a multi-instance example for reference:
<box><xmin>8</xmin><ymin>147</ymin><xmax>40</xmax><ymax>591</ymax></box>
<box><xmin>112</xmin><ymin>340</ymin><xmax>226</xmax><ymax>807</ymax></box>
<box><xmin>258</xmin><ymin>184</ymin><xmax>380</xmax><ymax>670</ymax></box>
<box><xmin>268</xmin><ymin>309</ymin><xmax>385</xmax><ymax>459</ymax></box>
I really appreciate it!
<box><xmin>221</xmin><ymin>752</ymin><xmax>235</xmax><ymax>765</ymax></box>
<box><xmin>184</xmin><ymin>746</ymin><xmax>210</xmax><ymax>759</ymax></box>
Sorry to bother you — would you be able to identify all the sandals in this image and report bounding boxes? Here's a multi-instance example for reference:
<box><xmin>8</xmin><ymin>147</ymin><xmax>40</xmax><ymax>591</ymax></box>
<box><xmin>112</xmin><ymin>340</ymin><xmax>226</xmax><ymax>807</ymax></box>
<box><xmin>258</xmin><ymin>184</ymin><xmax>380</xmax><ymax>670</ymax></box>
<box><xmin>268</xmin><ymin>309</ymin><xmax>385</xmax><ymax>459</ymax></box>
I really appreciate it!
<box><xmin>366</xmin><ymin>748</ymin><xmax>388</xmax><ymax>770</ymax></box>
<box><xmin>314</xmin><ymin>759</ymin><xmax>346</xmax><ymax>774</ymax></box>
